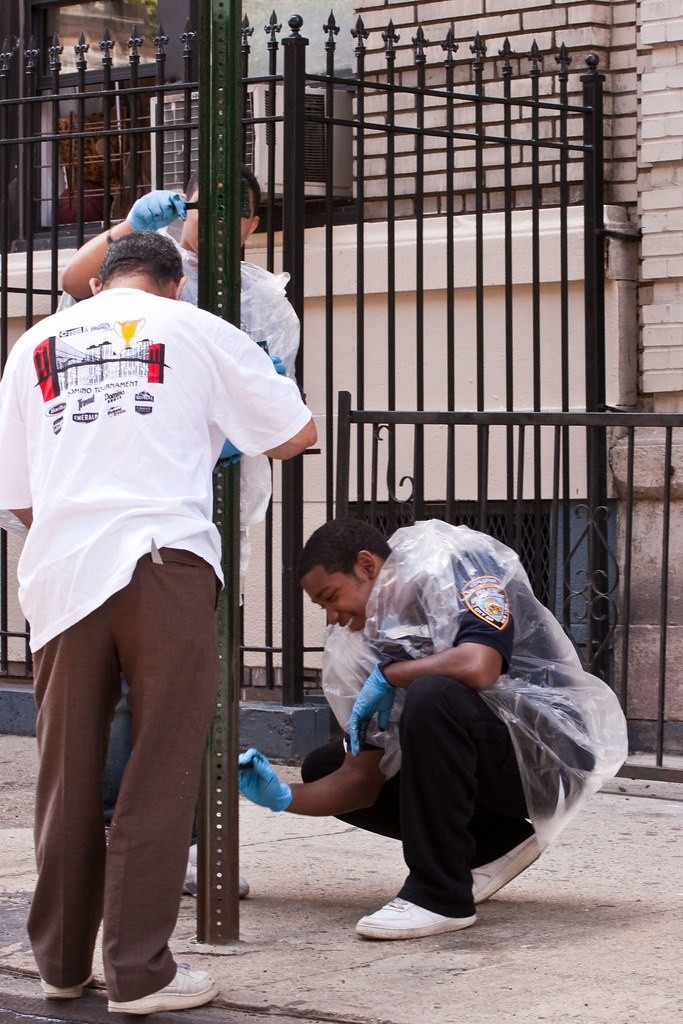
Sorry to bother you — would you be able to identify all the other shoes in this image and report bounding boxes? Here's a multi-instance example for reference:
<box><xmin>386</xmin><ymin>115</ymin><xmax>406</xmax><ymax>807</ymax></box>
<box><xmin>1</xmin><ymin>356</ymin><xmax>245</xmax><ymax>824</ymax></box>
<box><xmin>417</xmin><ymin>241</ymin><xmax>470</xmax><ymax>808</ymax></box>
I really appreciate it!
<box><xmin>182</xmin><ymin>848</ymin><xmax>250</xmax><ymax>897</ymax></box>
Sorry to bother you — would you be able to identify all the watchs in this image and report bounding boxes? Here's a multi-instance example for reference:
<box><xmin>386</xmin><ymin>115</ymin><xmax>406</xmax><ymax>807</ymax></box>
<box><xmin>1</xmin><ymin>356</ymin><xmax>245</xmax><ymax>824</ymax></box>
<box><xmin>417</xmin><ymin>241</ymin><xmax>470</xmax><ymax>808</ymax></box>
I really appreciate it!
<box><xmin>379</xmin><ymin>657</ymin><xmax>399</xmax><ymax>688</ymax></box>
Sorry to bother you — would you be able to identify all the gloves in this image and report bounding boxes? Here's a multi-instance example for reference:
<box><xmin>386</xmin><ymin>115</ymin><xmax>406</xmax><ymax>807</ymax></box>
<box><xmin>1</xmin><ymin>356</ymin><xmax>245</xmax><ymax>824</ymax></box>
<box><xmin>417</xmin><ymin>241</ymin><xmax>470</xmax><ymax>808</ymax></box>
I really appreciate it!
<box><xmin>270</xmin><ymin>356</ymin><xmax>286</xmax><ymax>376</ymax></box>
<box><xmin>238</xmin><ymin>748</ymin><xmax>292</xmax><ymax>812</ymax></box>
<box><xmin>215</xmin><ymin>440</ymin><xmax>241</xmax><ymax>471</ymax></box>
<box><xmin>127</xmin><ymin>189</ymin><xmax>186</xmax><ymax>232</ymax></box>
<box><xmin>349</xmin><ymin>661</ymin><xmax>396</xmax><ymax>756</ymax></box>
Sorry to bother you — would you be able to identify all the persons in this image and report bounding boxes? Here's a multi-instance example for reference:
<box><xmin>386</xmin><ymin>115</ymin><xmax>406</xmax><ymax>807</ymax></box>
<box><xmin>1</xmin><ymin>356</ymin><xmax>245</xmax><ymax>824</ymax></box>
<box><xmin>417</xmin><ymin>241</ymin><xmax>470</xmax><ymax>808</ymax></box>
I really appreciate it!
<box><xmin>239</xmin><ymin>518</ymin><xmax>598</xmax><ymax>942</ymax></box>
<box><xmin>1</xmin><ymin>231</ymin><xmax>319</xmax><ymax>1015</ymax></box>
<box><xmin>60</xmin><ymin>164</ymin><xmax>302</xmax><ymax>897</ymax></box>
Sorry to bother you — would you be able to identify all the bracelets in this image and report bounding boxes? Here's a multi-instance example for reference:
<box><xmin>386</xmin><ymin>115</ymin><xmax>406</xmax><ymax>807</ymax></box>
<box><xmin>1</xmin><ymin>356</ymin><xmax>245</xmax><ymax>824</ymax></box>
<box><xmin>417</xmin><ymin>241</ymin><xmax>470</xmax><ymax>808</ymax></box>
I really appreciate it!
<box><xmin>105</xmin><ymin>228</ymin><xmax>114</xmax><ymax>246</ymax></box>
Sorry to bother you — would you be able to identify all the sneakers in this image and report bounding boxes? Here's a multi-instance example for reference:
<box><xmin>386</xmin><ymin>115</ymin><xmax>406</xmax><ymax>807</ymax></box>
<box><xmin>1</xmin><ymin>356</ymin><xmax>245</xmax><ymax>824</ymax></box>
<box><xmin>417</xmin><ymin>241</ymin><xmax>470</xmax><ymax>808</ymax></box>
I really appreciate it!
<box><xmin>40</xmin><ymin>971</ymin><xmax>93</xmax><ymax>999</ymax></box>
<box><xmin>470</xmin><ymin>833</ymin><xmax>546</xmax><ymax>904</ymax></box>
<box><xmin>106</xmin><ymin>962</ymin><xmax>218</xmax><ymax>1016</ymax></box>
<box><xmin>356</xmin><ymin>898</ymin><xmax>476</xmax><ymax>938</ymax></box>
